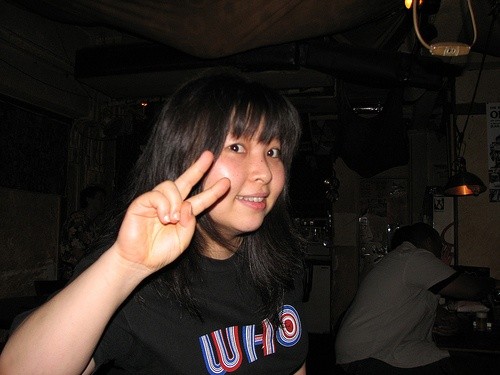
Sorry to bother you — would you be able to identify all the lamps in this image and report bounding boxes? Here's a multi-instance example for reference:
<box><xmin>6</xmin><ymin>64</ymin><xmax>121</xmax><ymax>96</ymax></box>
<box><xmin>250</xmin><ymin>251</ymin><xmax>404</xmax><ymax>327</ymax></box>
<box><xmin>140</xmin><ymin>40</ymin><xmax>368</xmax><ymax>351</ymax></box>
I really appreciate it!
<box><xmin>443</xmin><ymin>156</ymin><xmax>488</xmax><ymax>198</ymax></box>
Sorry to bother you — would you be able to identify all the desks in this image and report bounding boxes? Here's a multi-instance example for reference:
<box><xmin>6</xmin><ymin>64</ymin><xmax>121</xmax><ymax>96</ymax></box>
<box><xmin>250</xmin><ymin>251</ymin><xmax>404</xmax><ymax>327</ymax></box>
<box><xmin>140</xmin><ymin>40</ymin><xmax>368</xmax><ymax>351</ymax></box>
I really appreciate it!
<box><xmin>434</xmin><ymin>308</ymin><xmax>500</xmax><ymax>375</ymax></box>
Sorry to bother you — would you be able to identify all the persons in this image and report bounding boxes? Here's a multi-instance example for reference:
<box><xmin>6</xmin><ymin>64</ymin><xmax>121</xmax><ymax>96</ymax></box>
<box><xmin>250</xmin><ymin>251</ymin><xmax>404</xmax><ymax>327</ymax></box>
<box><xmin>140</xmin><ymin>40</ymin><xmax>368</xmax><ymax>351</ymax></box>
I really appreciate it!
<box><xmin>334</xmin><ymin>222</ymin><xmax>499</xmax><ymax>375</ymax></box>
<box><xmin>0</xmin><ymin>68</ymin><xmax>312</xmax><ymax>375</ymax></box>
<box><xmin>59</xmin><ymin>185</ymin><xmax>116</xmax><ymax>284</ymax></box>
